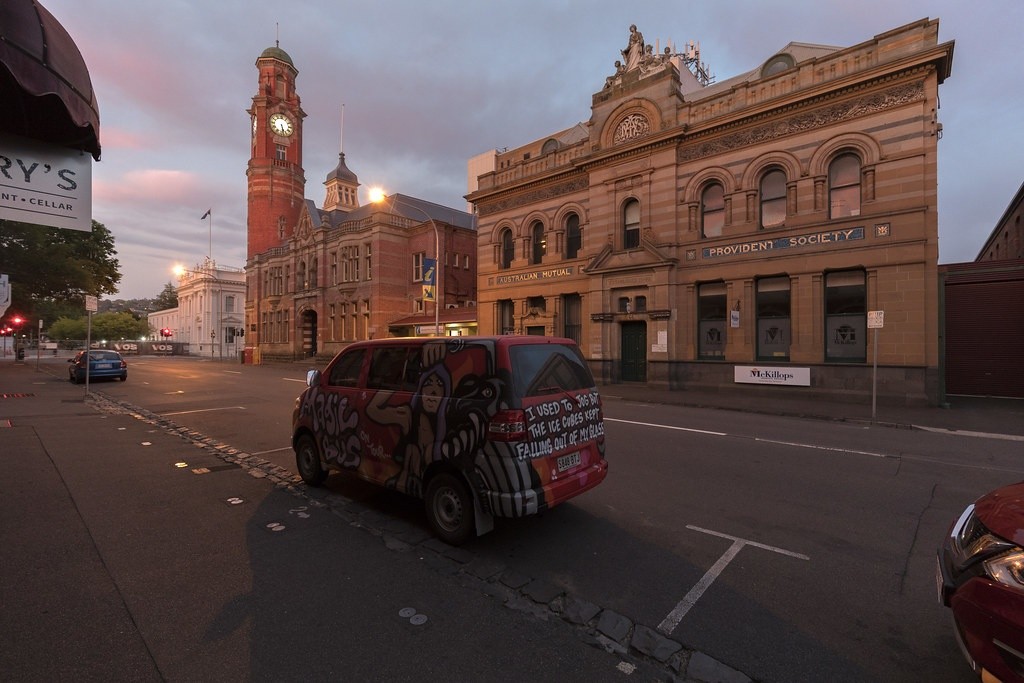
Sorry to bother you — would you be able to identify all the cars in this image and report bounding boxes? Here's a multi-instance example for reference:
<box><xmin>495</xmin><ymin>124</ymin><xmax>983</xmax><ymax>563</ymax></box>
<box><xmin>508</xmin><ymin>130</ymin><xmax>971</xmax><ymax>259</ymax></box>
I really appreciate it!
<box><xmin>67</xmin><ymin>350</ymin><xmax>128</xmax><ymax>385</ymax></box>
<box><xmin>936</xmin><ymin>480</ymin><xmax>1024</xmax><ymax>683</ymax></box>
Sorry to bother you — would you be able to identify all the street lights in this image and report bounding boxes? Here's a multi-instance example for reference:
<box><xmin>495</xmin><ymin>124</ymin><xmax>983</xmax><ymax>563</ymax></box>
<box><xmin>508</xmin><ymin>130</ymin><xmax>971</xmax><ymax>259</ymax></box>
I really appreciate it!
<box><xmin>174</xmin><ymin>268</ymin><xmax>222</xmax><ymax>358</ymax></box>
<box><xmin>370</xmin><ymin>190</ymin><xmax>439</xmax><ymax>336</ymax></box>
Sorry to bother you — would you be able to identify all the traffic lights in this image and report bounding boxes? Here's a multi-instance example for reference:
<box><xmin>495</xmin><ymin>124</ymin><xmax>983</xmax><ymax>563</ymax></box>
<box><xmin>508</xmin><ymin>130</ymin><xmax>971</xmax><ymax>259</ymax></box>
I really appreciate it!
<box><xmin>159</xmin><ymin>329</ymin><xmax>172</xmax><ymax>337</ymax></box>
<box><xmin>237</xmin><ymin>331</ymin><xmax>239</xmax><ymax>336</ymax></box>
<box><xmin>0</xmin><ymin>327</ymin><xmax>11</xmax><ymax>334</ymax></box>
<box><xmin>14</xmin><ymin>317</ymin><xmax>22</xmax><ymax>330</ymax></box>
<box><xmin>240</xmin><ymin>328</ymin><xmax>244</xmax><ymax>338</ymax></box>
<box><xmin>212</xmin><ymin>333</ymin><xmax>215</xmax><ymax>338</ymax></box>
<box><xmin>232</xmin><ymin>328</ymin><xmax>235</xmax><ymax>336</ymax></box>
<box><xmin>14</xmin><ymin>332</ymin><xmax>16</xmax><ymax>337</ymax></box>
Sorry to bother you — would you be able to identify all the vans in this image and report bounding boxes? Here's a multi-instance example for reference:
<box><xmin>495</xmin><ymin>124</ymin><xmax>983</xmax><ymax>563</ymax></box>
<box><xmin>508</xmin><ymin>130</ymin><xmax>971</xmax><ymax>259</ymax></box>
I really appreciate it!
<box><xmin>289</xmin><ymin>336</ymin><xmax>607</xmax><ymax>547</ymax></box>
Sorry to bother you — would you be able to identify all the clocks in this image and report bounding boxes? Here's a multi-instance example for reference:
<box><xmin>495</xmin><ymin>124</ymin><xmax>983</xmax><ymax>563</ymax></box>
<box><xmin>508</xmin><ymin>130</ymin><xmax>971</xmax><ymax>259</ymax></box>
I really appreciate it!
<box><xmin>269</xmin><ymin>112</ymin><xmax>294</xmax><ymax>137</ymax></box>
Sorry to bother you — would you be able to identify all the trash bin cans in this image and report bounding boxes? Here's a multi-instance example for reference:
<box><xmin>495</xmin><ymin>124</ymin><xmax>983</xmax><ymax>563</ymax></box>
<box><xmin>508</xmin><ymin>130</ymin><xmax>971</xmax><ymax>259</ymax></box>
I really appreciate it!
<box><xmin>238</xmin><ymin>351</ymin><xmax>244</xmax><ymax>363</ymax></box>
<box><xmin>244</xmin><ymin>347</ymin><xmax>263</xmax><ymax>365</ymax></box>
<box><xmin>15</xmin><ymin>344</ymin><xmax>25</xmax><ymax>360</ymax></box>
